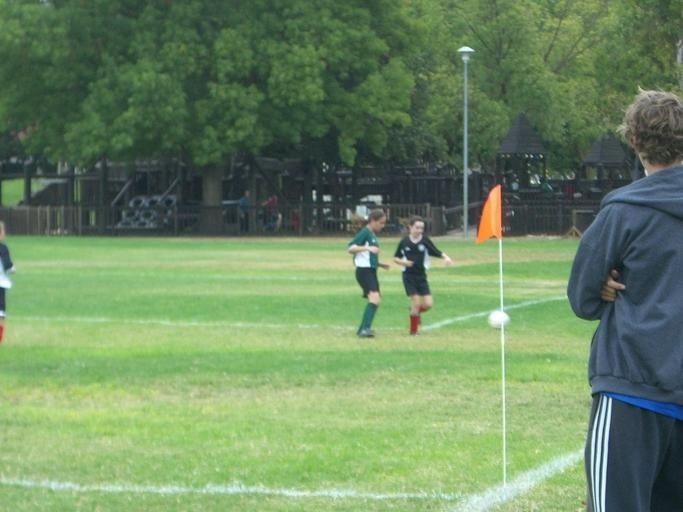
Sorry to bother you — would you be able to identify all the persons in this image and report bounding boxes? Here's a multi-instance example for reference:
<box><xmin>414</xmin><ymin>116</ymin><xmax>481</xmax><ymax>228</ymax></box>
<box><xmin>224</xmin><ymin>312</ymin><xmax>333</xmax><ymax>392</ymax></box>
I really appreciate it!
<box><xmin>346</xmin><ymin>210</ymin><xmax>390</xmax><ymax>337</ymax></box>
<box><xmin>0</xmin><ymin>219</ymin><xmax>12</xmax><ymax>347</ymax></box>
<box><xmin>567</xmin><ymin>87</ymin><xmax>682</xmax><ymax>512</ymax></box>
<box><xmin>391</xmin><ymin>215</ymin><xmax>453</xmax><ymax>336</ymax></box>
<box><xmin>237</xmin><ymin>189</ymin><xmax>249</xmax><ymax>233</ymax></box>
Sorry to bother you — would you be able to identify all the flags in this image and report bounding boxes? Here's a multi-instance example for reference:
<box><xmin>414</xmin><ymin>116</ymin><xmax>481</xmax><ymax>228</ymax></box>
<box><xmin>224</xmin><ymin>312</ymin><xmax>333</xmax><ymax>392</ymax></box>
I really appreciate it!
<box><xmin>473</xmin><ymin>186</ymin><xmax>501</xmax><ymax>245</ymax></box>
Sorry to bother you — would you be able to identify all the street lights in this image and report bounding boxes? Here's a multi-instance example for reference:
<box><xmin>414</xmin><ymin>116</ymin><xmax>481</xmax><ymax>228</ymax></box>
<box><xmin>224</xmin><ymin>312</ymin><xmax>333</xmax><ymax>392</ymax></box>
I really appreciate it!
<box><xmin>458</xmin><ymin>44</ymin><xmax>475</xmax><ymax>233</ymax></box>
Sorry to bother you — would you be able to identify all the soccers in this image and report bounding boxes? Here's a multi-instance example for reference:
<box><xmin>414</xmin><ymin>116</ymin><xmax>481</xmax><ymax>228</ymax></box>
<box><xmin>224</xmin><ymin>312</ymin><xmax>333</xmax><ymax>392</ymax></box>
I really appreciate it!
<box><xmin>488</xmin><ymin>309</ymin><xmax>510</xmax><ymax>330</ymax></box>
